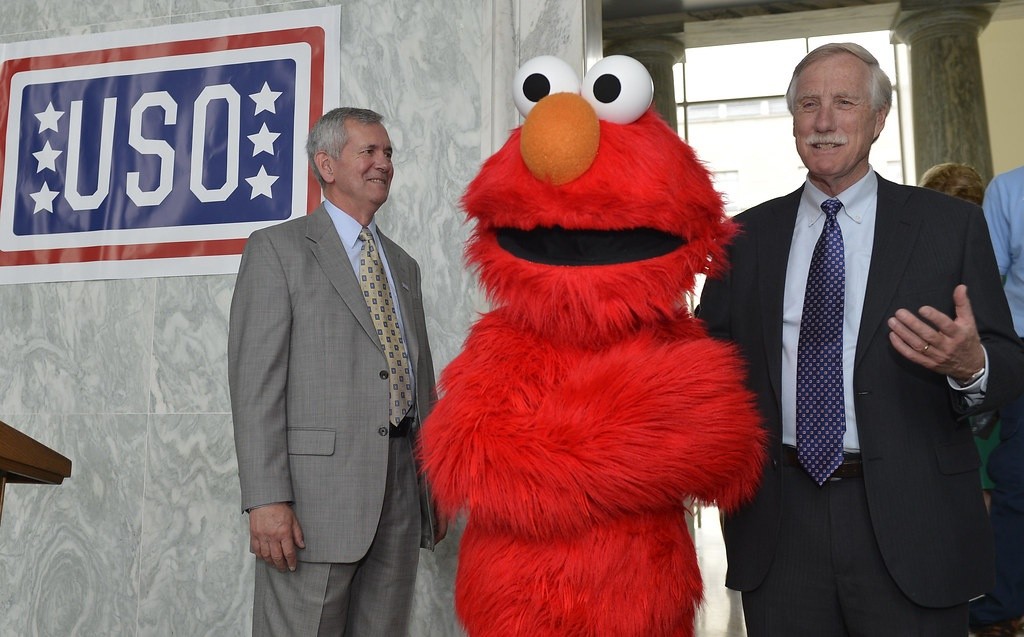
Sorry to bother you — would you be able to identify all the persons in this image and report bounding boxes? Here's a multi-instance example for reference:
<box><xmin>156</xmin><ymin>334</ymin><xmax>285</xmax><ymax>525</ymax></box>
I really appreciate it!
<box><xmin>227</xmin><ymin>107</ymin><xmax>448</xmax><ymax>637</ymax></box>
<box><xmin>697</xmin><ymin>43</ymin><xmax>1024</xmax><ymax>637</ymax></box>
<box><xmin>917</xmin><ymin>163</ymin><xmax>1024</xmax><ymax>637</ymax></box>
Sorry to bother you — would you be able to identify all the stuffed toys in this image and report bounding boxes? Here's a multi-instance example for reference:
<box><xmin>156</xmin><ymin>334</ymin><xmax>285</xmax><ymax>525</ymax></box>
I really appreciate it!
<box><xmin>416</xmin><ymin>56</ymin><xmax>767</xmax><ymax>637</ymax></box>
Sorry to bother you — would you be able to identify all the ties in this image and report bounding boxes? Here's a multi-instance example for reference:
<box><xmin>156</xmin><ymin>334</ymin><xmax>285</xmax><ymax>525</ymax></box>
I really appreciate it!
<box><xmin>357</xmin><ymin>226</ymin><xmax>413</xmax><ymax>428</ymax></box>
<box><xmin>796</xmin><ymin>197</ymin><xmax>847</xmax><ymax>483</ymax></box>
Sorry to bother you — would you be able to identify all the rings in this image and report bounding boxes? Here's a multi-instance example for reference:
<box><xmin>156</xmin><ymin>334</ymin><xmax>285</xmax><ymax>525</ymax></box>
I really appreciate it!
<box><xmin>922</xmin><ymin>344</ymin><xmax>929</xmax><ymax>353</ymax></box>
